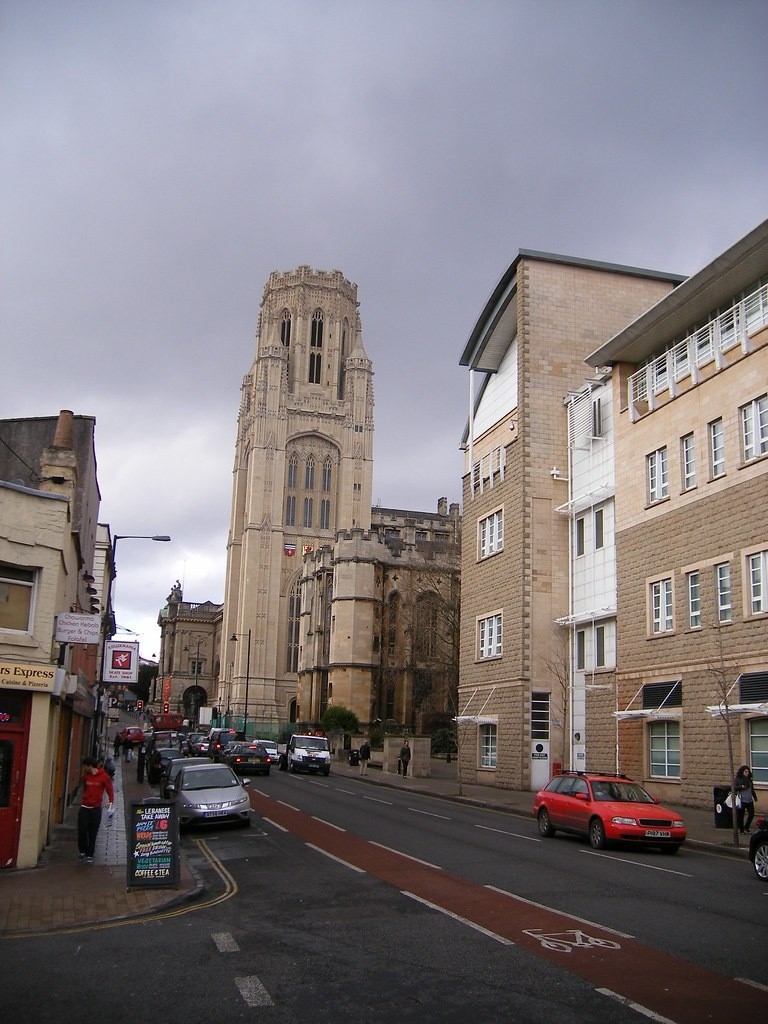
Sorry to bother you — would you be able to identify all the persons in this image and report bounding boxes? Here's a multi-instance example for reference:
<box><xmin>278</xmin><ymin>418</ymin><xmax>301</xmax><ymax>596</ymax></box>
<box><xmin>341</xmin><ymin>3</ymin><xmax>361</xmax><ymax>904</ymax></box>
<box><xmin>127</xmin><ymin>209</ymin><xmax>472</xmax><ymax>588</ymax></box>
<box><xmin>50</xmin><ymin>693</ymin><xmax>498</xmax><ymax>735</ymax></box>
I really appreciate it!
<box><xmin>77</xmin><ymin>757</ymin><xmax>114</xmax><ymax>862</ymax></box>
<box><xmin>181</xmin><ymin>717</ymin><xmax>192</xmax><ymax>734</ymax></box>
<box><xmin>358</xmin><ymin>740</ymin><xmax>371</xmax><ymax>776</ymax></box>
<box><xmin>104</xmin><ymin>731</ymin><xmax>133</xmax><ymax>779</ymax></box>
<box><xmin>397</xmin><ymin>740</ymin><xmax>411</xmax><ymax>778</ymax></box>
<box><xmin>734</xmin><ymin>765</ymin><xmax>759</xmax><ymax>836</ymax></box>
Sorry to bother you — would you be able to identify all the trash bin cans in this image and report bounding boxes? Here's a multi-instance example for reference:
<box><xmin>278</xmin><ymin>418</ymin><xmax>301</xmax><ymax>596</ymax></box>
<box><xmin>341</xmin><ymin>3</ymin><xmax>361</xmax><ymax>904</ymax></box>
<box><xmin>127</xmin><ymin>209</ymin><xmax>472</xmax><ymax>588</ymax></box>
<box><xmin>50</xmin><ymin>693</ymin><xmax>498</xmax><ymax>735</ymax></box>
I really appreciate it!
<box><xmin>348</xmin><ymin>749</ymin><xmax>359</xmax><ymax>766</ymax></box>
<box><xmin>713</xmin><ymin>784</ymin><xmax>746</xmax><ymax>829</ymax></box>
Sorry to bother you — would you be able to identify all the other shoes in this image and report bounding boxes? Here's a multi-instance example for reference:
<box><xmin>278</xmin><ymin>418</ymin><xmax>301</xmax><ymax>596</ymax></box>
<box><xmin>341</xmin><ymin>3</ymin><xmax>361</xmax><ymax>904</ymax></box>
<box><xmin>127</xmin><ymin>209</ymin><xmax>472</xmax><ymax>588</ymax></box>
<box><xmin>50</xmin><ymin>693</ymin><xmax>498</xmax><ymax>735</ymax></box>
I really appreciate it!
<box><xmin>741</xmin><ymin>832</ymin><xmax>745</xmax><ymax>835</ymax></box>
<box><xmin>403</xmin><ymin>776</ymin><xmax>406</xmax><ymax>779</ymax></box>
<box><xmin>86</xmin><ymin>856</ymin><xmax>93</xmax><ymax>862</ymax></box>
<box><xmin>113</xmin><ymin>754</ymin><xmax>119</xmax><ymax>757</ymax></box>
<box><xmin>364</xmin><ymin>774</ymin><xmax>368</xmax><ymax>776</ymax></box>
<box><xmin>744</xmin><ymin>827</ymin><xmax>750</xmax><ymax>832</ymax></box>
<box><xmin>125</xmin><ymin>760</ymin><xmax>130</xmax><ymax>763</ymax></box>
<box><xmin>79</xmin><ymin>853</ymin><xmax>85</xmax><ymax>860</ymax></box>
<box><xmin>360</xmin><ymin>774</ymin><xmax>363</xmax><ymax>775</ymax></box>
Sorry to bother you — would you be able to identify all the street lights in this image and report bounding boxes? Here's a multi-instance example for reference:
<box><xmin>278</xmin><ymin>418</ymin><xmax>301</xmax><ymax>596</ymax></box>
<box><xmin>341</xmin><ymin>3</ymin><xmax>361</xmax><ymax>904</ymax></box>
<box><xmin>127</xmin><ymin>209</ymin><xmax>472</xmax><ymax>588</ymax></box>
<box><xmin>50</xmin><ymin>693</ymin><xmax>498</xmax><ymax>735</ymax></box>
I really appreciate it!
<box><xmin>92</xmin><ymin>535</ymin><xmax>172</xmax><ymax>764</ymax></box>
<box><xmin>151</xmin><ymin>647</ymin><xmax>165</xmax><ymax>714</ymax></box>
<box><xmin>229</xmin><ymin>629</ymin><xmax>251</xmax><ymax>734</ymax></box>
<box><xmin>183</xmin><ymin>639</ymin><xmax>199</xmax><ymax>685</ymax></box>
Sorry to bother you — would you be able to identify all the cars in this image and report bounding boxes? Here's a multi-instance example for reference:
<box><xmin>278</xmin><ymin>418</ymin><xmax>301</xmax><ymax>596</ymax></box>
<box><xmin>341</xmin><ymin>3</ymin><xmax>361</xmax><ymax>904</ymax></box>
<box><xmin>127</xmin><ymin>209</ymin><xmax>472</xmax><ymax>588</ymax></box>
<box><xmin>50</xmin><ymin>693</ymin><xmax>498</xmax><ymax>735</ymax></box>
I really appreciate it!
<box><xmin>225</xmin><ymin>744</ymin><xmax>271</xmax><ymax>776</ymax></box>
<box><xmin>747</xmin><ymin>813</ymin><xmax>768</xmax><ymax>881</ymax></box>
<box><xmin>160</xmin><ymin>757</ymin><xmax>213</xmax><ymax>801</ymax></box>
<box><xmin>252</xmin><ymin>739</ymin><xmax>279</xmax><ymax>765</ymax></box>
<box><xmin>532</xmin><ymin>769</ymin><xmax>687</xmax><ymax>856</ymax></box>
<box><xmin>119</xmin><ymin>726</ymin><xmax>252</xmax><ymax>784</ymax></box>
<box><xmin>166</xmin><ymin>764</ymin><xmax>252</xmax><ymax>833</ymax></box>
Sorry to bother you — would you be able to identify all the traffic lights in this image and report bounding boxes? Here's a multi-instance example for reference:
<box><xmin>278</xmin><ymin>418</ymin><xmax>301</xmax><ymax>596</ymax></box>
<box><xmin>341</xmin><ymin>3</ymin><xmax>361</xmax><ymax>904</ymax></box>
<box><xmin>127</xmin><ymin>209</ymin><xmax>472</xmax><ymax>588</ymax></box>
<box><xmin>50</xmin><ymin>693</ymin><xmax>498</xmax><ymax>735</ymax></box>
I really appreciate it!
<box><xmin>163</xmin><ymin>702</ymin><xmax>170</xmax><ymax>714</ymax></box>
<box><xmin>137</xmin><ymin>700</ymin><xmax>143</xmax><ymax>710</ymax></box>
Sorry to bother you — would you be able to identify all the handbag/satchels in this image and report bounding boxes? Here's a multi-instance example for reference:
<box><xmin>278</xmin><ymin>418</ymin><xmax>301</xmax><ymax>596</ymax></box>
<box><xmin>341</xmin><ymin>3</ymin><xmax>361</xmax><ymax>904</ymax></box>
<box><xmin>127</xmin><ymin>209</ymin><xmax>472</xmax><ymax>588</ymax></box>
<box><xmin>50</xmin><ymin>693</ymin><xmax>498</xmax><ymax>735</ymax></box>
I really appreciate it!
<box><xmin>724</xmin><ymin>789</ymin><xmax>742</xmax><ymax>808</ymax></box>
<box><xmin>104</xmin><ymin>804</ymin><xmax>114</xmax><ymax>828</ymax></box>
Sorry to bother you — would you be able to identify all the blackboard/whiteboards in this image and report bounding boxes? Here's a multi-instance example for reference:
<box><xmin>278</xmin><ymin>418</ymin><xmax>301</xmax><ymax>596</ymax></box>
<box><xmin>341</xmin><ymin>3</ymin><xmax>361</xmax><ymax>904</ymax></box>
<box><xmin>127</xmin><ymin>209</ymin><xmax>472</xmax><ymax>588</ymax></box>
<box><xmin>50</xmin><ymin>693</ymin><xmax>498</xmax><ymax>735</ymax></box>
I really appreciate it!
<box><xmin>124</xmin><ymin>800</ymin><xmax>183</xmax><ymax>889</ymax></box>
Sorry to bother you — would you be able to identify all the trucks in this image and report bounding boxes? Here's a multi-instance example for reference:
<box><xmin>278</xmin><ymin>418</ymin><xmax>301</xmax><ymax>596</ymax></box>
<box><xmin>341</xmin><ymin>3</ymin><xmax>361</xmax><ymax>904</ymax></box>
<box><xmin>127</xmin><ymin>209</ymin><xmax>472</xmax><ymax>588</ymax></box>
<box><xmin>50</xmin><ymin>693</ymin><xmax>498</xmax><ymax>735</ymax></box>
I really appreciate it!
<box><xmin>277</xmin><ymin>733</ymin><xmax>335</xmax><ymax>776</ymax></box>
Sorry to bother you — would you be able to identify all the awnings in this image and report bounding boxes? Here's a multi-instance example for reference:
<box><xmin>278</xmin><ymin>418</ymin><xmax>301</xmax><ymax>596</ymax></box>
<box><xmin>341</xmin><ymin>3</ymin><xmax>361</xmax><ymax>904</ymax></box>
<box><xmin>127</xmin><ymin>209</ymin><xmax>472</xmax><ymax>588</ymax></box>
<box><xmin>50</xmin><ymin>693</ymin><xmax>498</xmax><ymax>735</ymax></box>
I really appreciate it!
<box><xmin>704</xmin><ymin>673</ymin><xmax>768</xmax><ymax>717</ymax></box>
<box><xmin>453</xmin><ymin>687</ymin><xmax>499</xmax><ymax>725</ymax></box>
<box><xmin>611</xmin><ymin>680</ymin><xmax>683</xmax><ymax>720</ymax></box>
